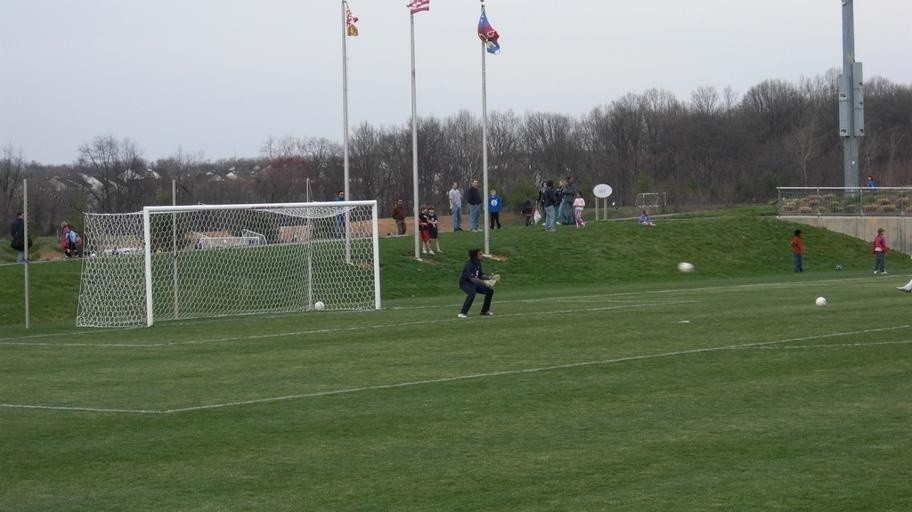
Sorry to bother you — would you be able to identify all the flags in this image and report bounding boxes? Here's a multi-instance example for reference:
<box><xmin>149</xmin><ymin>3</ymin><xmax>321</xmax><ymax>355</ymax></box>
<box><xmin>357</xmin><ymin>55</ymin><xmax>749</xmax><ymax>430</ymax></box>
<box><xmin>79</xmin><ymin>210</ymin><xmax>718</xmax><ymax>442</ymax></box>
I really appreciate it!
<box><xmin>405</xmin><ymin>0</ymin><xmax>429</xmax><ymax>12</ymax></box>
<box><xmin>345</xmin><ymin>3</ymin><xmax>358</xmax><ymax>36</ymax></box>
<box><xmin>478</xmin><ymin>5</ymin><xmax>499</xmax><ymax>55</ymax></box>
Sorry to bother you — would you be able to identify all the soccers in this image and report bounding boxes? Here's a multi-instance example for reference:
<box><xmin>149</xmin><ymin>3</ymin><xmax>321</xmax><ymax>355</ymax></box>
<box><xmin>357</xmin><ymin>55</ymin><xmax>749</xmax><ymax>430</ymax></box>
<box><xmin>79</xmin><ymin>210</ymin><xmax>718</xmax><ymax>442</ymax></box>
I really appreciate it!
<box><xmin>314</xmin><ymin>302</ymin><xmax>324</xmax><ymax>311</ymax></box>
<box><xmin>816</xmin><ymin>296</ymin><xmax>826</xmax><ymax>306</ymax></box>
<box><xmin>678</xmin><ymin>262</ymin><xmax>693</xmax><ymax>273</ymax></box>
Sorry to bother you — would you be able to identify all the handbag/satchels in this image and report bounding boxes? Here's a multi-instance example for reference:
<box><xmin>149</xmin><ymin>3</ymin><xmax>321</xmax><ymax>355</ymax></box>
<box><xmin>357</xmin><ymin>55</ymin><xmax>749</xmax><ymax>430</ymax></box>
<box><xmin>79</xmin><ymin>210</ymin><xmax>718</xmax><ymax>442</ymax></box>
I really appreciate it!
<box><xmin>534</xmin><ymin>206</ymin><xmax>541</xmax><ymax>224</ymax></box>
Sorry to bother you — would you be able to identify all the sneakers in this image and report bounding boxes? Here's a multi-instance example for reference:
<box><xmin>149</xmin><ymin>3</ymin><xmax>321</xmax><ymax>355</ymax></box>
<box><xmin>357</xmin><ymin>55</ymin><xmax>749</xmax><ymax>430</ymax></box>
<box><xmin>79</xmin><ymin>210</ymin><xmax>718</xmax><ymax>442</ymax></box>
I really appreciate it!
<box><xmin>422</xmin><ymin>249</ymin><xmax>443</xmax><ymax>255</ymax></box>
<box><xmin>880</xmin><ymin>271</ymin><xmax>888</xmax><ymax>275</ymax></box>
<box><xmin>542</xmin><ymin>220</ymin><xmax>586</xmax><ymax>233</ymax></box>
<box><xmin>896</xmin><ymin>286</ymin><xmax>911</xmax><ymax>292</ymax></box>
<box><xmin>479</xmin><ymin>311</ymin><xmax>493</xmax><ymax>315</ymax></box>
<box><xmin>458</xmin><ymin>313</ymin><xmax>468</xmax><ymax>318</ymax></box>
<box><xmin>453</xmin><ymin>224</ymin><xmax>502</xmax><ymax>232</ymax></box>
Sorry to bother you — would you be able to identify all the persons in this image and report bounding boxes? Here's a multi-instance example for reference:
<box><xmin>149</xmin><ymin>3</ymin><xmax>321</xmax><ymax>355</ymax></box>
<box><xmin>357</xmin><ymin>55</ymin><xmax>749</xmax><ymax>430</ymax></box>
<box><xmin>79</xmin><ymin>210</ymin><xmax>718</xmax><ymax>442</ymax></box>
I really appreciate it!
<box><xmin>466</xmin><ymin>180</ymin><xmax>483</xmax><ymax>232</ymax></box>
<box><xmin>333</xmin><ymin>190</ymin><xmax>346</xmax><ymax>239</ymax></box>
<box><xmin>60</xmin><ymin>220</ymin><xmax>82</xmax><ymax>257</ymax></box>
<box><xmin>11</xmin><ymin>212</ymin><xmax>32</xmax><ymax>263</ymax></box>
<box><xmin>522</xmin><ymin>176</ymin><xmax>585</xmax><ymax>232</ymax></box>
<box><xmin>872</xmin><ymin>228</ymin><xmax>888</xmax><ymax>275</ymax></box>
<box><xmin>489</xmin><ymin>187</ymin><xmax>503</xmax><ymax>229</ymax></box>
<box><xmin>790</xmin><ymin>230</ymin><xmax>804</xmax><ymax>272</ymax></box>
<box><xmin>639</xmin><ymin>208</ymin><xmax>656</xmax><ymax>226</ymax></box>
<box><xmin>867</xmin><ymin>176</ymin><xmax>879</xmax><ymax>187</ymax></box>
<box><xmin>456</xmin><ymin>249</ymin><xmax>500</xmax><ymax>318</ymax></box>
<box><xmin>449</xmin><ymin>181</ymin><xmax>464</xmax><ymax>231</ymax></box>
<box><xmin>392</xmin><ymin>199</ymin><xmax>443</xmax><ymax>256</ymax></box>
